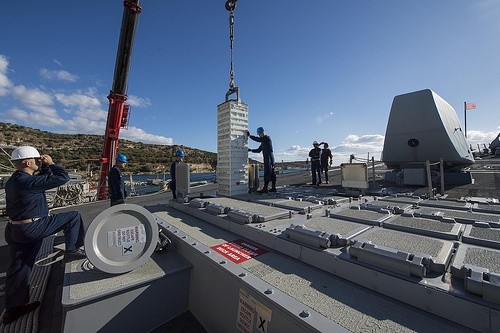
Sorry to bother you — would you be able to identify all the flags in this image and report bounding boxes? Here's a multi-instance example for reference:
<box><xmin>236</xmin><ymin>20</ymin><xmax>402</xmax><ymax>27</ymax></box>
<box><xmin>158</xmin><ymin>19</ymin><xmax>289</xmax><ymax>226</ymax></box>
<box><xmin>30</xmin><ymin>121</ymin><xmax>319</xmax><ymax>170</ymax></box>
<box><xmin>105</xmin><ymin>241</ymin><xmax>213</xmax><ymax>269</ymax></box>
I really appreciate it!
<box><xmin>466</xmin><ymin>103</ymin><xmax>476</xmax><ymax>110</ymax></box>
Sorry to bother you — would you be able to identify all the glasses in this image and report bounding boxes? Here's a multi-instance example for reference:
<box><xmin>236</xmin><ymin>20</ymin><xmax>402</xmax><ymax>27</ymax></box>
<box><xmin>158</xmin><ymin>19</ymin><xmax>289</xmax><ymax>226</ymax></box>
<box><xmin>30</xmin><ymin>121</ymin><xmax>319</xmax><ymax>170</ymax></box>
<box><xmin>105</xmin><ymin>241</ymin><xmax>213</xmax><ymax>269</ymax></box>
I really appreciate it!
<box><xmin>259</xmin><ymin>134</ymin><xmax>260</xmax><ymax>136</ymax></box>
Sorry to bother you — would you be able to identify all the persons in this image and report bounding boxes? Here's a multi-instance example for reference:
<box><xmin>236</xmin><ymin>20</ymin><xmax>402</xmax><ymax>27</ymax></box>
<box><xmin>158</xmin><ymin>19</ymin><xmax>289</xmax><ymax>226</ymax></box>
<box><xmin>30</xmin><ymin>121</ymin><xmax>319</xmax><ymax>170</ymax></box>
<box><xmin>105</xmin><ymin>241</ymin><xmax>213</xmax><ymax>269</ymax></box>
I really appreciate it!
<box><xmin>3</xmin><ymin>146</ymin><xmax>87</xmax><ymax>323</ymax></box>
<box><xmin>320</xmin><ymin>143</ymin><xmax>334</xmax><ymax>184</ymax></box>
<box><xmin>248</xmin><ymin>127</ymin><xmax>277</xmax><ymax>193</ymax></box>
<box><xmin>170</xmin><ymin>150</ymin><xmax>186</xmax><ymax>199</ymax></box>
<box><xmin>108</xmin><ymin>153</ymin><xmax>128</xmax><ymax>207</ymax></box>
<box><xmin>309</xmin><ymin>141</ymin><xmax>322</xmax><ymax>186</ymax></box>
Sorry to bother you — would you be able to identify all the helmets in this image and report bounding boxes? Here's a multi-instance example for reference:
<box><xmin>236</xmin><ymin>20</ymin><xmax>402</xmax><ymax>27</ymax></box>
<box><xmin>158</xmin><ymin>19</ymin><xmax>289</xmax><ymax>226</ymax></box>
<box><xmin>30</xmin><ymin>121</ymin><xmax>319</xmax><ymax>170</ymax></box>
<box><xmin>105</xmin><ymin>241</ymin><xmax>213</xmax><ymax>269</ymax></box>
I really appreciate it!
<box><xmin>313</xmin><ymin>141</ymin><xmax>320</xmax><ymax>145</ymax></box>
<box><xmin>116</xmin><ymin>153</ymin><xmax>128</xmax><ymax>162</ymax></box>
<box><xmin>176</xmin><ymin>149</ymin><xmax>184</xmax><ymax>156</ymax></box>
<box><xmin>256</xmin><ymin>127</ymin><xmax>264</xmax><ymax>134</ymax></box>
<box><xmin>11</xmin><ymin>146</ymin><xmax>41</xmax><ymax>160</ymax></box>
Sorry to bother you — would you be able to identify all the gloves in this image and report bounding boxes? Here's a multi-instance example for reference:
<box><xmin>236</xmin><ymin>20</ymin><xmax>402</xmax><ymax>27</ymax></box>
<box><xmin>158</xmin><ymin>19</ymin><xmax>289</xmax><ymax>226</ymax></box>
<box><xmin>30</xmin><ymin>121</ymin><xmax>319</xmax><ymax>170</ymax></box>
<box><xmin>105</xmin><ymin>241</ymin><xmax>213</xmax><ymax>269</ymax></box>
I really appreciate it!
<box><xmin>247</xmin><ymin>131</ymin><xmax>250</xmax><ymax>136</ymax></box>
<box><xmin>248</xmin><ymin>149</ymin><xmax>251</xmax><ymax>151</ymax></box>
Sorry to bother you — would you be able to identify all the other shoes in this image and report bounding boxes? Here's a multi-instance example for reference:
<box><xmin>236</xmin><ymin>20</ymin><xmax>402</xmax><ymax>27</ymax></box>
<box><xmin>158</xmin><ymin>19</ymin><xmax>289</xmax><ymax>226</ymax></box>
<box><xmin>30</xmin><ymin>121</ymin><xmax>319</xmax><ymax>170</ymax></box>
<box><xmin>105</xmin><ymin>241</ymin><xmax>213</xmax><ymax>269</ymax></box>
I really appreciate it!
<box><xmin>269</xmin><ymin>188</ymin><xmax>276</xmax><ymax>192</ymax></box>
<box><xmin>327</xmin><ymin>181</ymin><xmax>329</xmax><ymax>184</ymax></box>
<box><xmin>64</xmin><ymin>249</ymin><xmax>86</xmax><ymax>262</ymax></box>
<box><xmin>257</xmin><ymin>189</ymin><xmax>268</xmax><ymax>192</ymax></box>
<box><xmin>4</xmin><ymin>299</ymin><xmax>41</xmax><ymax>324</ymax></box>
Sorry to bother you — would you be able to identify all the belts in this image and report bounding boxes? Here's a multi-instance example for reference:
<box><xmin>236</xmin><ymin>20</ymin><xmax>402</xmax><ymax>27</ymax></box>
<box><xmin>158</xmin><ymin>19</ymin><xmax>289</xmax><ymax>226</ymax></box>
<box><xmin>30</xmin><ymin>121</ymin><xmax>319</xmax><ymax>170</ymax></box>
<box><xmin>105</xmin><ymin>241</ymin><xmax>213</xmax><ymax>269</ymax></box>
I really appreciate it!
<box><xmin>10</xmin><ymin>217</ymin><xmax>39</xmax><ymax>224</ymax></box>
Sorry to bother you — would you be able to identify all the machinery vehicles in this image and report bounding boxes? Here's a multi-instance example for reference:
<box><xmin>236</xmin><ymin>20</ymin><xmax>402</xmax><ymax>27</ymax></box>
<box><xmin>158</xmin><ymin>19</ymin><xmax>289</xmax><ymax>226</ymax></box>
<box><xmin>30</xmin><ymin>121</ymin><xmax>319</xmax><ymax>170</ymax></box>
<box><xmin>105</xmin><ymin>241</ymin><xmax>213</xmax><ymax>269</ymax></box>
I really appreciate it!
<box><xmin>96</xmin><ymin>0</ymin><xmax>238</xmax><ymax>202</ymax></box>
